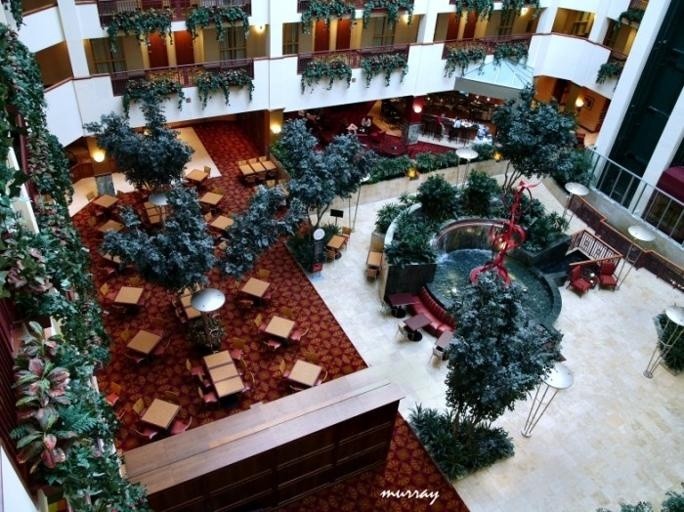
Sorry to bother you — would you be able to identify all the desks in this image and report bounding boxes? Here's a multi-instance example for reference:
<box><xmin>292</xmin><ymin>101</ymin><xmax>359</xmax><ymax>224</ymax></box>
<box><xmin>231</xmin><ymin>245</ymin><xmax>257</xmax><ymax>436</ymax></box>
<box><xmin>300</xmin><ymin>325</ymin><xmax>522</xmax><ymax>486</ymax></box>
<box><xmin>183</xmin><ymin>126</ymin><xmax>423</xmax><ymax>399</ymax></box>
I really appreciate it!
<box><xmin>404</xmin><ymin>313</ymin><xmax>432</xmax><ymax>342</ymax></box>
<box><xmin>434</xmin><ymin>331</ymin><xmax>454</xmax><ymax>350</ymax></box>
<box><xmin>388</xmin><ymin>294</ymin><xmax>415</xmax><ymax>318</ymax></box>
<box><xmin>368</xmin><ymin>251</ymin><xmax>382</xmax><ymax>270</ymax></box>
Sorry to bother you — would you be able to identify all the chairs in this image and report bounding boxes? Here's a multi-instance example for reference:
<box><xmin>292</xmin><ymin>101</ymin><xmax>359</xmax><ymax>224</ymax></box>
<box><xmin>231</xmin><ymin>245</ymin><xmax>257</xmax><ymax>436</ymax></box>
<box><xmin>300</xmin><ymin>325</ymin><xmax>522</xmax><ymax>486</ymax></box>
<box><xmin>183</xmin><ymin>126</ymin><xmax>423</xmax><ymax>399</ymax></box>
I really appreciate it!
<box><xmin>324</xmin><ymin>227</ymin><xmax>351</xmax><ymax>261</ymax></box>
<box><xmin>97</xmin><ymin>269</ymin><xmax>330</xmax><ymax>439</ymax></box>
<box><xmin>395</xmin><ymin>323</ymin><xmax>407</xmax><ymax>341</ymax></box>
<box><xmin>429</xmin><ymin>348</ymin><xmax>444</xmax><ymax>366</ymax></box>
<box><xmin>357</xmin><ymin>114</ymin><xmax>386</xmax><ymax>143</ymax></box>
<box><xmin>566</xmin><ymin>259</ymin><xmax>619</xmax><ymax>295</ymax></box>
<box><xmin>379</xmin><ymin>297</ymin><xmax>392</xmax><ymax>317</ymax></box>
<box><xmin>80</xmin><ymin>155</ymin><xmax>287</xmax><ymax>239</ymax></box>
